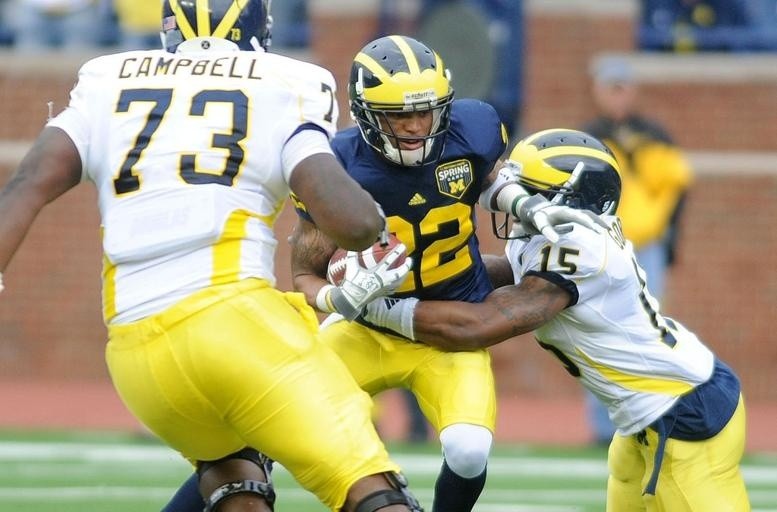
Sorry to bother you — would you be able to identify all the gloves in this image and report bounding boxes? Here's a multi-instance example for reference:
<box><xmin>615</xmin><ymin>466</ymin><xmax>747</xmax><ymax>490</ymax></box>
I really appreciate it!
<box><xmin>331</xmin><ymin>242</ymin><xmax>413</xmax><ymax>322</ymax></box>
<box><xmin>519</xmin><ymin>193</ymin><xmax>611</xmax><ymax>243</ymax></box>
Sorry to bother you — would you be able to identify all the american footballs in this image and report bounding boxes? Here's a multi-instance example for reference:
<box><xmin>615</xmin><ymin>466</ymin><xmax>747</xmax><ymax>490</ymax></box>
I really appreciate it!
<box><xmin>326</xmin><ymin>234</ymin><xmax>406</xmax><ymax>286</ymax></box>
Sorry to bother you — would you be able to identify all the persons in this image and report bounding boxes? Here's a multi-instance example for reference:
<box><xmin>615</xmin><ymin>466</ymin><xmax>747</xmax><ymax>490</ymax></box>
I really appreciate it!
<box><xmin>0</xmin><ymin>0</ymin><xmax>755</xmax><ymax>512</ymax></box>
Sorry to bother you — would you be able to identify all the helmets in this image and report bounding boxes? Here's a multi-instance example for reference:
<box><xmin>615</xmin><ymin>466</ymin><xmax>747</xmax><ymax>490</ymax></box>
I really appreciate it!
<box><xmin>159</xmin><ymin>0</ymin><xmax>273</xmax><ymax>49</ymax></box>
<box><xmin>491</xmin><ymin>128</ymin><xmax>622</xmax><ymax>240</ymax></box>
<box><xmin>347</xmin><ymin>35</ymin><xmax>455</xmax><ymax>164</ymax></box>
